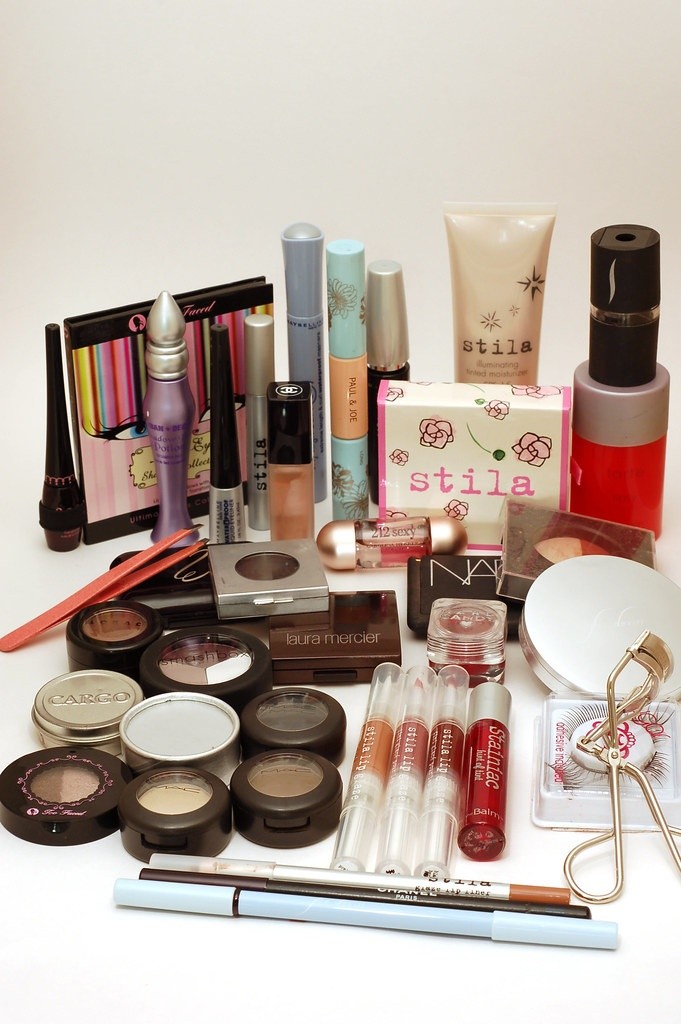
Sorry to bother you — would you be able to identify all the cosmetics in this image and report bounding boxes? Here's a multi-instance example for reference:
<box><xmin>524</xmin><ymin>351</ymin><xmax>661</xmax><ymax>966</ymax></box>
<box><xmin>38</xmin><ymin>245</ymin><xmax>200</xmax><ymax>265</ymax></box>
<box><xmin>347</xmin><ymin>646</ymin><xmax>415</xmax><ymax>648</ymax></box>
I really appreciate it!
<box><xmin>0</xmin><ymin>201</ymin><xmax>677</xmax><ymax>874</ymax></box>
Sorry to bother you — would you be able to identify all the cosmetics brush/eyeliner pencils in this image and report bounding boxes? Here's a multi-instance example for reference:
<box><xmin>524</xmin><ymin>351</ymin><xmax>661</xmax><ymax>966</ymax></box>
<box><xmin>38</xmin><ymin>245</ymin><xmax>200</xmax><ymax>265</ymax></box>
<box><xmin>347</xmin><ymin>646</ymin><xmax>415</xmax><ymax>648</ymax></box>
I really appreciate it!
<box><xmin>111</xmin><ymin>853</ymin><xmax>620</xmax><ymax>950</ymax></box>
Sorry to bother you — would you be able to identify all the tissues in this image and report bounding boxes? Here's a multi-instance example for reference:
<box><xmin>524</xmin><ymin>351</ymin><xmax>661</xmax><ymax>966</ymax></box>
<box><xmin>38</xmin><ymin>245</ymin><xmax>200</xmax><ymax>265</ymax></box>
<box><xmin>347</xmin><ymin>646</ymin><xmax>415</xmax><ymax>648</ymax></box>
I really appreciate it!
<box><xmin>375</xmin><ymin>379</ymin><xmax>573</xmax><ymax>546</ymax></box>
